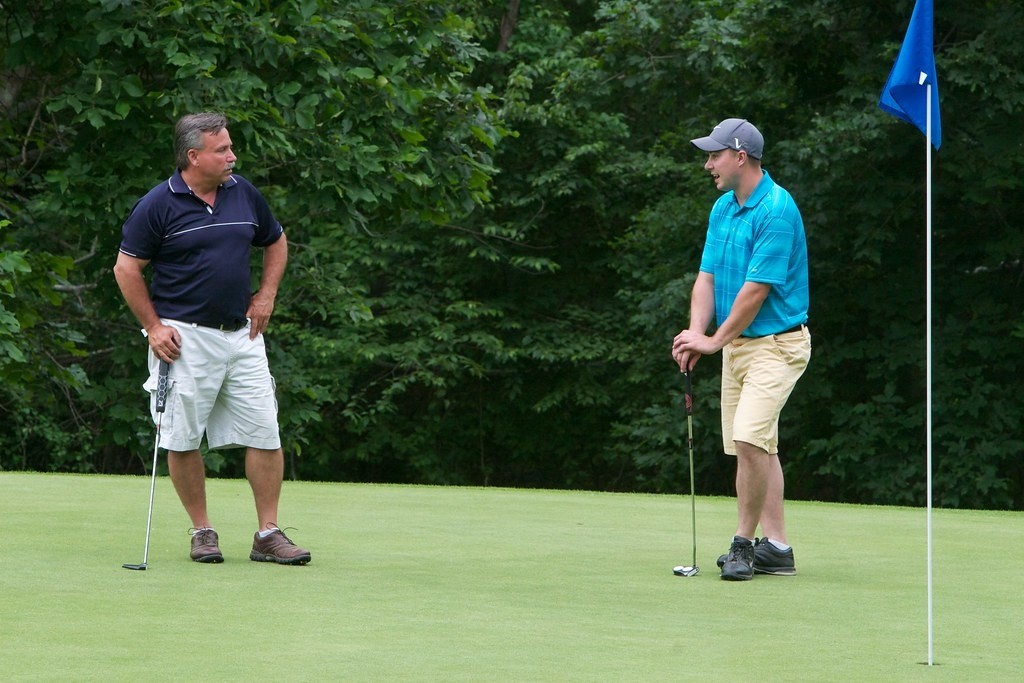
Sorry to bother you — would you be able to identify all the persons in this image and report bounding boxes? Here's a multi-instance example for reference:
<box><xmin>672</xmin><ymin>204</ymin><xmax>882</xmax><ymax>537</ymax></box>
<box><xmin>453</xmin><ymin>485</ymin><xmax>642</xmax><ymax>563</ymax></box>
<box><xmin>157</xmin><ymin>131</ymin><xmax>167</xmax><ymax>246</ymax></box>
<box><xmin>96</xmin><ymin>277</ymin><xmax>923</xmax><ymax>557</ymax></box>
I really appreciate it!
<box><xmin>113</xmin><ymin>112</ymin><xmax>310</xmax><ymax>565</ymax></box>
<box><xmin>672</xmin><ymin>118</ymin><xmax>812</xmax><ymax>580</ymax></box>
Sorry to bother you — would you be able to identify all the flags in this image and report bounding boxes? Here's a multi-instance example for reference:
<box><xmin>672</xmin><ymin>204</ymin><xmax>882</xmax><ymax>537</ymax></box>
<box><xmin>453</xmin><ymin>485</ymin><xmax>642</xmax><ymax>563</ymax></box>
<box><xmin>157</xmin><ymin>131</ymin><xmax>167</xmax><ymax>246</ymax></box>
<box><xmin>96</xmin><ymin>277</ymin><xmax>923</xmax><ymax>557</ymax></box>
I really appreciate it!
<box><xmin>878</xmin><ymin>0</ymin><xmax>942</xmax><ymax>153</ymax></box>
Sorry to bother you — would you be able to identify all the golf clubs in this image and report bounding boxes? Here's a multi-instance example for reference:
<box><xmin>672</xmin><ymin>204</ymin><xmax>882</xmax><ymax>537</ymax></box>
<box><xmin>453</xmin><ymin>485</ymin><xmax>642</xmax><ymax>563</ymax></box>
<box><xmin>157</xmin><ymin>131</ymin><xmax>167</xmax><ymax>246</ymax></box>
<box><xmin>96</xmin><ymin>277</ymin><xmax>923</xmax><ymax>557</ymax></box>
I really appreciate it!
<box><xmin>122</xmin><ymin>357</ymin><xmax>169</xmax><ymax>570</ymax></box>
<box><xmin>672</xmin><ymin>358</ymin><xmax>700</xmax><ymax>577</ymax></box>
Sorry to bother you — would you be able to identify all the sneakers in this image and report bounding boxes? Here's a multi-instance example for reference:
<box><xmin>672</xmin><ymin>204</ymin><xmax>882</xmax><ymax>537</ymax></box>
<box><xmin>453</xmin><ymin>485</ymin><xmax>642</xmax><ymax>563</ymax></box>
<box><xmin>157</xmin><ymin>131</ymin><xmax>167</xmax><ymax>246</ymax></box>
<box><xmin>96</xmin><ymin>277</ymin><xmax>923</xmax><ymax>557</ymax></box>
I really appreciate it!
<box><xmin>721</xmin><ymin>535</ymin><xmax>755</xmax><ymax>580</ymax></box>
<box><xmin>187</xmin><ymin>524</ymin><xmax>223</xmax><ymax>562</ymax></box>
<box><xmin>718</xmin><ymin>535</ymin><xmax>797</xmax><ymax>575</ymax></box>
<box><xmin>249</xmin><ymin>521</ymin><xmax>311</xmax><ymax>564</ymax></box>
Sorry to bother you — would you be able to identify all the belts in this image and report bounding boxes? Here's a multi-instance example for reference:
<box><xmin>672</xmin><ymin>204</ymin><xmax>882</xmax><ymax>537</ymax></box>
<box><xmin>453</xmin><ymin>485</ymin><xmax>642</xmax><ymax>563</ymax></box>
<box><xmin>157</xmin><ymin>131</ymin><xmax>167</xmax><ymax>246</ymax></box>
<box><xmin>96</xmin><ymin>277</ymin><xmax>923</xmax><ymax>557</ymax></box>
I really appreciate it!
<box><xmin>190</xmin><ymin>321</ymin><xmax>248</xmax><ymax>331</ymax></box>
<box><xmin>738</xmin><ymin>323</ymin><xmax>806</xmax><ymax>338</ymax></box>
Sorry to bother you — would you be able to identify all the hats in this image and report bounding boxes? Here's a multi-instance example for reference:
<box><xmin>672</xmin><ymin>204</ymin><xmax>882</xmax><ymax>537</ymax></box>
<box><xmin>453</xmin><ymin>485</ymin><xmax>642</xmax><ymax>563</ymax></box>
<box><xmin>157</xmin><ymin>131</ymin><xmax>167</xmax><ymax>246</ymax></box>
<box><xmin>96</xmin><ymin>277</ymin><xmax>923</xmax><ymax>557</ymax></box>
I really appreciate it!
<box><xmin>690</xmin><ymin>118</ymin><xmax>763</xmax><ymax>160</ymax></box>
